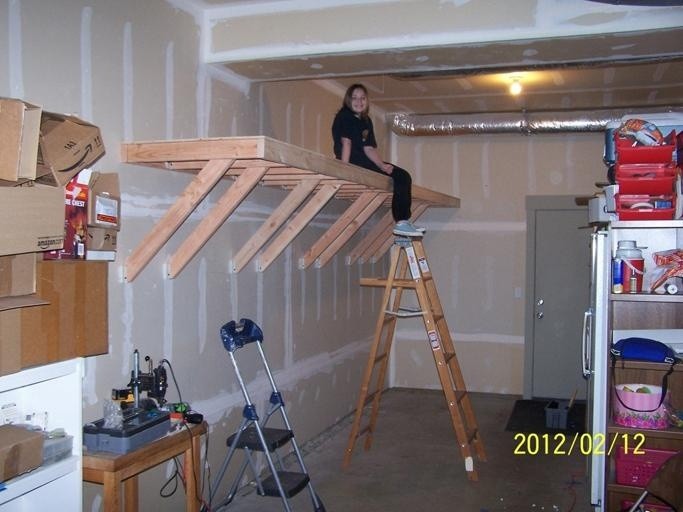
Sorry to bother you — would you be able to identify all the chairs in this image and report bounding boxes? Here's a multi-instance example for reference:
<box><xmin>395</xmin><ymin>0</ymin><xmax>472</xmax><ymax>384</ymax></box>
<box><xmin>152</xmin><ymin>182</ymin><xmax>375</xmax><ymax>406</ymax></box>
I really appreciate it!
<box><xmin>628</xmin><ymin>452</ymin><xmax>683</xmax><ymax>512</ymax></box>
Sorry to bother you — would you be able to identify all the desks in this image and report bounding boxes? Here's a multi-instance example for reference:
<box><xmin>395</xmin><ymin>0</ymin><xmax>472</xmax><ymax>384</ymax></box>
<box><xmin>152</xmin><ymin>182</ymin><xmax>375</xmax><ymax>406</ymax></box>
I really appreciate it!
<box><xmin>83</xmin><ymin>417</ymin><xmax>209</xmax><ymax>512</ymax></box>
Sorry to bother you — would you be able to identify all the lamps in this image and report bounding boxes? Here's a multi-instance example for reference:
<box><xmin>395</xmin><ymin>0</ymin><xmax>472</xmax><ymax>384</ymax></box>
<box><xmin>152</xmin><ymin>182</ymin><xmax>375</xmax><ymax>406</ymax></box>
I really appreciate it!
<box><xmin>510</xmin><ymin>79</ymin><xmax>521</xmax><ymax>95</ymax></box>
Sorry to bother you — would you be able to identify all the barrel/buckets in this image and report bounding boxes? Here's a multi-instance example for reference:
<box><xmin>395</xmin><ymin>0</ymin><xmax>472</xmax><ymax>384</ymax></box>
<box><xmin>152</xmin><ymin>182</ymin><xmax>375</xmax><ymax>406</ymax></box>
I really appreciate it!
<box><xmin>616</xmin><ymin>239</ymin><xmax>646</xmax><ymax>292</ymax></box>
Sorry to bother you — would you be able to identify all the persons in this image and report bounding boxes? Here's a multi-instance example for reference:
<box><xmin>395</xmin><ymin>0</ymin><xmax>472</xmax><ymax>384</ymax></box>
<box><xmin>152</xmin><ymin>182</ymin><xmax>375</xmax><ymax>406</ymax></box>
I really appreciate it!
<box><xmin>330</xmin><ymin>83</ymin><xmax>428</xmax><ymax>238</ymax></box>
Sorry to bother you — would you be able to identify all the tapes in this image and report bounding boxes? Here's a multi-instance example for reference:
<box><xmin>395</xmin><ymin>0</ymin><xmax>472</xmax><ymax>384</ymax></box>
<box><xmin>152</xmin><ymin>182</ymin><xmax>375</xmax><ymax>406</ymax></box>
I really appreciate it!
<box><xmin>630</xmin><ymin>202</ymin><xmax>655</xmax><ymax>210</ymax></box>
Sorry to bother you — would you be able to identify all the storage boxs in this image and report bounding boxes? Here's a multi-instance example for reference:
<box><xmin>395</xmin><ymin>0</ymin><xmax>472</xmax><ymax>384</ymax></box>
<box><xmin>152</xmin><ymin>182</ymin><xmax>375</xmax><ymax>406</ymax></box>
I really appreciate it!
<box><xmin>544</xmin><ymin>397</ymin><xmax>575</xmax><ymax>429</ymax></box>
<box><xmin>587</xmin><ymin>128</ymin><xmax>677</xmax><ymax>224</ymax></box>
<box><xmin>0</xmin><ymin>424</ymin><xmax>42</xmax><ymax>483</ymax></box>
<box><xmin>0</xmin><ymin>96</ymin><xmax>121</xmax><ymax>374</ymax></box>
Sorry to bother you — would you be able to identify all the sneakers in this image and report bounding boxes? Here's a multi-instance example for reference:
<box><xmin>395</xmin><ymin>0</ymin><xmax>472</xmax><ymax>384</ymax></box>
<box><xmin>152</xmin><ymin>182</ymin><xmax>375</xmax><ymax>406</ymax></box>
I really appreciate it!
<box><xmin>393</xmin><ymin>219</ymin><xmax>426</xmax><ymax>236</ymax></box>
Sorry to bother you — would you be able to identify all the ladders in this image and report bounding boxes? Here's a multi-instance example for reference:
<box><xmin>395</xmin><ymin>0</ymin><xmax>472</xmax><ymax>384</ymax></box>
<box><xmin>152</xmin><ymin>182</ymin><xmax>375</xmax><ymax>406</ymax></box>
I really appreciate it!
<box><xmin>200</xmin><ymin>318</ymin><xmax>326</xmax><ymax>512</ymax></box>
<box><xmin>341</xmin><ymin>237</ymin><xmax>489</xmax><ymax>482</ymax></box>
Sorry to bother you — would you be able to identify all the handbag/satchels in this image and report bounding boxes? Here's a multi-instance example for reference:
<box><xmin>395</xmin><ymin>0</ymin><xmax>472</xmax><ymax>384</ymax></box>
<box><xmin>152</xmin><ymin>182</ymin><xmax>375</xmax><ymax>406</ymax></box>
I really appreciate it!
<box><xmin>616</xmin><ymin>447</ymin><xmax>681</xmax><ymax>488</ymax></box>
<box><xmin>611</xmin><ymin>338</ymin><xmax>673</xmax><ymax>364</ymax></box>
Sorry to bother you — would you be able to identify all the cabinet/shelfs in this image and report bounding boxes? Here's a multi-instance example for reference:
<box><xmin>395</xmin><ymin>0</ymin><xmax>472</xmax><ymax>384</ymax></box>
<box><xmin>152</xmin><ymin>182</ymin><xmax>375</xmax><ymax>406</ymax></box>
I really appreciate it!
<box><xmin>0</xmin><ymin>356</ymin><xmax>83</xmax><ymax>512</ymax></box>
<box><xmin>603</xmin><ymin>220</ymin><xmax>683</xmax><ymax>512</ymax></box>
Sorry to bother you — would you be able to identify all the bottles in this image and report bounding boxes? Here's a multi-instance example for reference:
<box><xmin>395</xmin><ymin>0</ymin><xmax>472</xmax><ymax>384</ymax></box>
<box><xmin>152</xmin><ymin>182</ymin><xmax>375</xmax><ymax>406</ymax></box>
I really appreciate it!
<box><xmin>630</xmin><ymin>266</ymin><xmax>637</xmax><ymax>295</ymax></box>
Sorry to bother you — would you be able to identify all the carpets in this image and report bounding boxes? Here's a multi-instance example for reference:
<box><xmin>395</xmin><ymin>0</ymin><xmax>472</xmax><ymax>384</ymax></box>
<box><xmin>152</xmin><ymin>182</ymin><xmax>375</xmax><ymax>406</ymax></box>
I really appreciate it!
<box><xmin>504</xmin><ymin>399</ymin><xmax>586</xmax><ymax>437</ymax></box>
